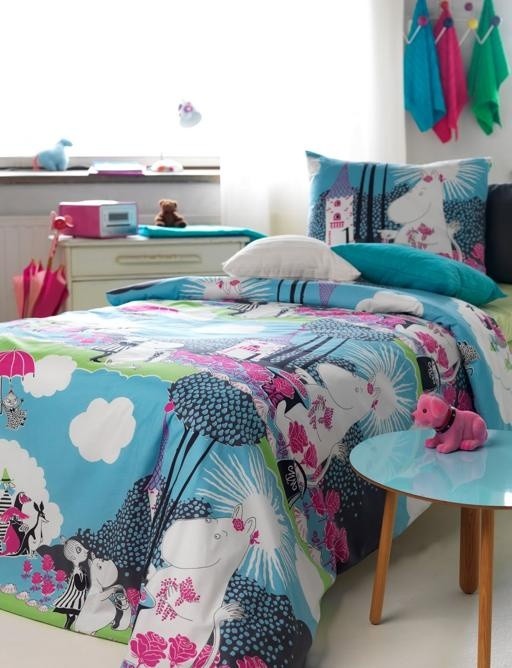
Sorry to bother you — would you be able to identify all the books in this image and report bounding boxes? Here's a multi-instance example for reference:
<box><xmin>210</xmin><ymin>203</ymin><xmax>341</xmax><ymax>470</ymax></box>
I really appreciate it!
<box><xmin>88</xmin><ymin>162</ymin><xmax>143</xmax><ymax>175</ymax></box>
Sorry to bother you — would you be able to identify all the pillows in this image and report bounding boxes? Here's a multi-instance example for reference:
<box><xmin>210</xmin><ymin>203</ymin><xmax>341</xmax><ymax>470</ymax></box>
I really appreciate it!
<box><xmin>484</xmin><ymin>182</ymin><xmax>512</xmax><ymax>285</ymax></box>
<box><xmin>223</xmin><ymin>235</ymin><xmax>360</xmax><ymax>282</ymax></box>
<box><xmin>305</xmin><ymin>150</ymin><xmax>492</xmax><ymax>279</ymax></box>
<box><xmin>331</xmin><ymin>242</ymin><xmax>508</xmax><ymax>305</ymax></box>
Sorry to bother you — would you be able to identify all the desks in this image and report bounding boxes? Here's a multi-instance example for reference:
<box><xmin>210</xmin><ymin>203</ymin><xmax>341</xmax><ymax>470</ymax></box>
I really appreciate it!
<box><xmin>349</xmin><ymin>426</ymin><xmax>512</xmax><ymax>668</ymax></box>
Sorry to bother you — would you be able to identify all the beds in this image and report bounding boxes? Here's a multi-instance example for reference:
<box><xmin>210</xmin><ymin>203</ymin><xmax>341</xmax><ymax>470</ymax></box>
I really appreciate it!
<box><xmin>0</xmin><ymin>287</ymin><xmax>511</xmax><ymax>668</ymax></box>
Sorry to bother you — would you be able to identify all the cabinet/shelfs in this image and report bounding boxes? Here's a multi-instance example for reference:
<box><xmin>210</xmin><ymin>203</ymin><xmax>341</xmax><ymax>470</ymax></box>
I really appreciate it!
<box><xmin>48</xmin><ymin>234</ymin><xmax>248</xmax><ymax>312</ymax></box>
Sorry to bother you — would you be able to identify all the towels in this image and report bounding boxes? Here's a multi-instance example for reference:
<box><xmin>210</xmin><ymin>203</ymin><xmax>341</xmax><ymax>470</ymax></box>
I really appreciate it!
<box><xmin>433</xmin><ymin>0</ymin><xmax>467</xmax><ymax>142</ymax></box>
<box><xmin>466</xmin><ymin>0</ymin><xmax>509</xmax><ymax>136</ymax></box>
<box><xmin>403</xmin><ymin>0</ymin><xmax>445</xmax><ymax>135</ymax></box>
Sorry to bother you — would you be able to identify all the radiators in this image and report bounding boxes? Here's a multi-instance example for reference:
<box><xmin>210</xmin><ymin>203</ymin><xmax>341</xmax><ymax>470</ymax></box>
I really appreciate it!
<box><xmin>0</xmin><ymin>215</ymin><xmax>62</xmax><ymax>322</ymax></box>
